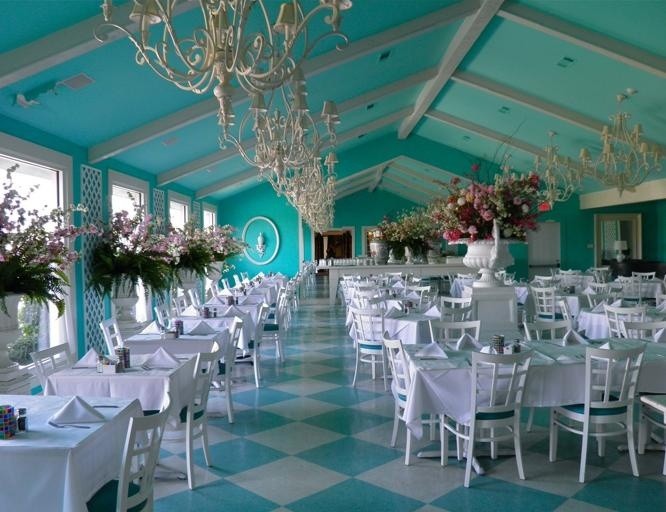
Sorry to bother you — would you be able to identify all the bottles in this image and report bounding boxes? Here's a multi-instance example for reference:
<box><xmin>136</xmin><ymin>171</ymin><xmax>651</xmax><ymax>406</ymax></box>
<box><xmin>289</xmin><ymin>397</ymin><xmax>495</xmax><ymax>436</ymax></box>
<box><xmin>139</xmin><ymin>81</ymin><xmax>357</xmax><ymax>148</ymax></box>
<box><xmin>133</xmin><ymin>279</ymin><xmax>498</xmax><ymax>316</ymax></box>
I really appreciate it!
<box><xmin>224</xmin><ymin>289</ymin><xmax>239</xmax><ymax>306</ymax></box>
<box><xmin>95</xmin><ymin>343</ymin><xmax>132</xmax><ymax>373</ymax></box>
<box><xmin>194</xmin><ymin>304</ymin><xmax>217</xmax><ymax>317</ymax></box>
<box><xmin>16</xmin><ymin>408</ymin><xmax>28</xmax><ymax>434</ymax></box>
<box><xmin>512</xmin><ymin>339</ymin><xmax>521</xmax><ymax>353</ymax></box>
<box><xmin>160</xmin><ymin>316</ymin><xmax>185</xmax><ymax>339</ymax></box>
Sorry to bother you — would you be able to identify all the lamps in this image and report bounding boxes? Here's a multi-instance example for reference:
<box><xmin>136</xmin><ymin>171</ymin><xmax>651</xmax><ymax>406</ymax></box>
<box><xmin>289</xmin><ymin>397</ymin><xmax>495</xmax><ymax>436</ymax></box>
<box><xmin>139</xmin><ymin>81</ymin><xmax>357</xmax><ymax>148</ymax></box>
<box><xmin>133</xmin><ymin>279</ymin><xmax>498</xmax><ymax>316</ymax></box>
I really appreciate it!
<box><xmin>574</xmin><ymin>82</ymin><xmax>666</xmax><ymax>197</ymax></box>
<box><xmin>532</xmin><ymin>129</ymin><xmax>583</xmax><ymax>207</ymax></box>
<box><xmin>100</xmin><ymin>0</ymin><xmax>353</xmax><ymax>241</ymax></box>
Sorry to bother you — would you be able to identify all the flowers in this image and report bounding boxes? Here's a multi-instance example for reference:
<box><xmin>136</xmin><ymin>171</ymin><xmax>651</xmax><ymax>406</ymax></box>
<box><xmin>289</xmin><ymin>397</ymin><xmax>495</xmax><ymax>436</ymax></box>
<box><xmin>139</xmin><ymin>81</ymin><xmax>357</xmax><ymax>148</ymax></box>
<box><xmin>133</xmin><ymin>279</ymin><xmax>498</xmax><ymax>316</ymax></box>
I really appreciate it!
<box><xmin>0</xmin><ymin>157</ymin><xmax>248</xmax><ymax>313</ymax></box>
<box><xmin>364</xmin><ymin>160</ymin><xmax>550</xmax><ymax>256</ymax></box>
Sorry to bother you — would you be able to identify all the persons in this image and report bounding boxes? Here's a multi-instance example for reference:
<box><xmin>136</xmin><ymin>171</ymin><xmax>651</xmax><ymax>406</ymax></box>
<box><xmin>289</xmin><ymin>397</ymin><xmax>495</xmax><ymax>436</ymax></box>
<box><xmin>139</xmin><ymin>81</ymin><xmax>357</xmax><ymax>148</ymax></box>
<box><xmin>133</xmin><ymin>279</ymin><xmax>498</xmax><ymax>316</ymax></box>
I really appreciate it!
<box><xmin>326</xmin><ymin>244</ymin><xmax>336</xmax><ymax>258</ymax></box>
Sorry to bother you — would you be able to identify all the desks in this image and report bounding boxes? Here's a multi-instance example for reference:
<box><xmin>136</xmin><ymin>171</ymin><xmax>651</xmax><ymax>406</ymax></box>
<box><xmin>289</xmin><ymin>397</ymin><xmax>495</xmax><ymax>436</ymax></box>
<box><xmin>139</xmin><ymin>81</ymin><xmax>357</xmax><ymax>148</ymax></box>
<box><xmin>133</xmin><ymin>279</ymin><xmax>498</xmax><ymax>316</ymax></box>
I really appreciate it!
<box><xmin>328</xmin><ymin>264</ymin><xmax>477</xmax><ymax>305</ymax></box>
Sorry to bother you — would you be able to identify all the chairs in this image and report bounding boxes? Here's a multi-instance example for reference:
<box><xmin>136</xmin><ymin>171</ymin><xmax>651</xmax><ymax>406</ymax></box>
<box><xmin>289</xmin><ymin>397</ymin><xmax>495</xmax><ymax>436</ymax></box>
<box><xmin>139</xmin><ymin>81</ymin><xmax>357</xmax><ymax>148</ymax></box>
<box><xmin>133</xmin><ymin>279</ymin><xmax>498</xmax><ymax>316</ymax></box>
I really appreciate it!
<box><xmin>1</xmin><ymin>317</ymin><xmax>244</xmax><ymax>512</ymax></box>
<box><xmin>153</xmin><ymin>262</ymin><xmax>316</xmax><ymax>388</ymax></box>
<box><xmin>340</xmin><ymin>267</ymin><xmax>666</xmax><ymax>488</ymax></box>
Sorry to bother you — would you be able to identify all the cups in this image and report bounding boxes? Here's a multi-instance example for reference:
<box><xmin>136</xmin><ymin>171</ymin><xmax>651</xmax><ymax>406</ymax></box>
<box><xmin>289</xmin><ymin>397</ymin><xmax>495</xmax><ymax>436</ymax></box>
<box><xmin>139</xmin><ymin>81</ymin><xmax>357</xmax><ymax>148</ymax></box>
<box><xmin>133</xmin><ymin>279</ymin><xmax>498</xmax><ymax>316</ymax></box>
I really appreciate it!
<box><xmin>317</xmin><ymin>257</ymin><xmax>374</xmax><ymax>266</ymax></box>
<box><xmin>504</xmin><ymin>272</ymin><xmax>529</xmax><ymax>287</ymax></box>
<box><xmin>489</xmin><ymin>333</ymin><xmax>504</xmax><ymax>355</ymax></box>
